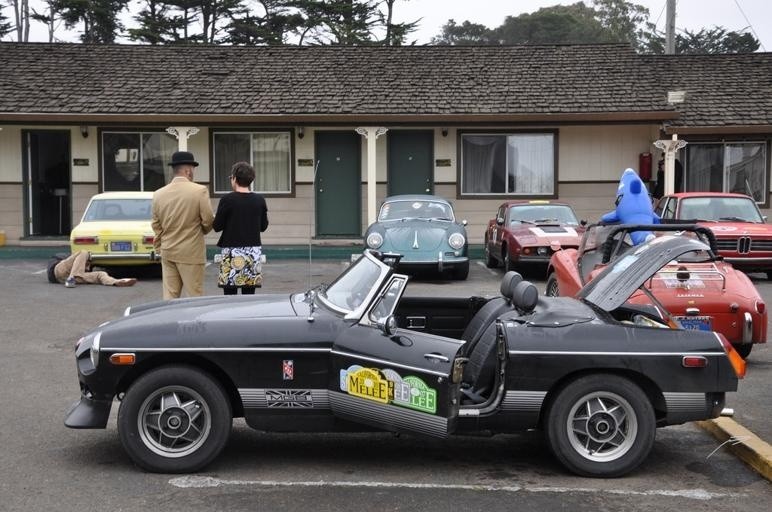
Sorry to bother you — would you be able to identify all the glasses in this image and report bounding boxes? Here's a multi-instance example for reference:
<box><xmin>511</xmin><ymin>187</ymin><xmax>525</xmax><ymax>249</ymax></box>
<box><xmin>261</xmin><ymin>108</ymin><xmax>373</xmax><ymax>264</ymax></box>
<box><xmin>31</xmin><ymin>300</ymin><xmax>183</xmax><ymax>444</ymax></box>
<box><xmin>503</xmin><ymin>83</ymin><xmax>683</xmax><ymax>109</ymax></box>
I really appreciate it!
<box><xmin>229</xmin><ymin>176</ymin><xmax>234</xmax><ymax>179</ymax></box>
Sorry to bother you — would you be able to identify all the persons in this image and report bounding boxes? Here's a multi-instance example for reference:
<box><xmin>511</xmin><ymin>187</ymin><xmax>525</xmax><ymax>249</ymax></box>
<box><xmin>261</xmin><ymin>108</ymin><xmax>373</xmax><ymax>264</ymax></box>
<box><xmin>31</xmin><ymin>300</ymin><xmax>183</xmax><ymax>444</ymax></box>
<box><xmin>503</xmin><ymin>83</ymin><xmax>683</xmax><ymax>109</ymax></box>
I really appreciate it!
<box><xmin>655</xmin><ymin>152</ymin><xmax>682</xmax><ymax>199</ymax></box>
<box><xmin>151</xmin><ymin>152</ymin><xmax>215</xmax><ymax>300</ymax></box>
<box><xmin>213</xmin><ymin>161</ymin><xmax>269</xmax><ymax>295</ymax></box>
<box><xmin>47</xmin><ymin>250</ymin><xmax>137</xmax><ymax>287</ymax></box>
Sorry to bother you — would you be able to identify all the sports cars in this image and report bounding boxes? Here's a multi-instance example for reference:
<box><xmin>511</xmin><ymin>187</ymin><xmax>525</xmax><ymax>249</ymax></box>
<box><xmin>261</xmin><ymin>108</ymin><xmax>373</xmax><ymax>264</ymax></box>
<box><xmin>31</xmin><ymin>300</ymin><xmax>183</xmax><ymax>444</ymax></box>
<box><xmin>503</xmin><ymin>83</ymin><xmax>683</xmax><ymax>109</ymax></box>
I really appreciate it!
<box><xmin>545</xmin><ymin>218</ymin><xmax>768</xmax><ymax>363</ymax></box>
<box><xmin>63</xmin><ymin>248</ymin><xmax>747</xmax><ymax>481</ymax></box>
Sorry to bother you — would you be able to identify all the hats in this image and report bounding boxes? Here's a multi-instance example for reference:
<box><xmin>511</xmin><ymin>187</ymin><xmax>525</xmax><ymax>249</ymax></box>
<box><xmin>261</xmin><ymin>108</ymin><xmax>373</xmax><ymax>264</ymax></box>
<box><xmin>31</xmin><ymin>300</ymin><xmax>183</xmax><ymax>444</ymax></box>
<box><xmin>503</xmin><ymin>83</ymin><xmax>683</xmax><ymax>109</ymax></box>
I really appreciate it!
<box><xmin>168</xmin><ymin>152</ymin><xmax>199</xmax><ymax>167</ymax></box>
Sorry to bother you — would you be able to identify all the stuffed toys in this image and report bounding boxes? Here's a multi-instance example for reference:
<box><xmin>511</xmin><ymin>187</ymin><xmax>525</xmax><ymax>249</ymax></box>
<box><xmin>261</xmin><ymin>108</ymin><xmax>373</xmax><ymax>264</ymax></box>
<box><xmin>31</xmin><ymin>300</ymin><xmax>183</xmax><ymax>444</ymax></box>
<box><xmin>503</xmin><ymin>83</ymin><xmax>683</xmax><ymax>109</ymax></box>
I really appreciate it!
<box><xmin>602</xmin><ymin>167</ymin><xmax>661</xmax><ymax>245</ymax></box>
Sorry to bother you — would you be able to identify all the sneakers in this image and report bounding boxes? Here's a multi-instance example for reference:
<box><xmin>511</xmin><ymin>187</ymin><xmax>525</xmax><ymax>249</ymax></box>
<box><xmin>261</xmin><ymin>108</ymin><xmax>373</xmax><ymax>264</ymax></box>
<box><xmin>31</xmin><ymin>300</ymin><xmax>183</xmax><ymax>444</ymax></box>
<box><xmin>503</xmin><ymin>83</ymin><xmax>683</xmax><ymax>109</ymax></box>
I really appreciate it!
<box><xmin>65</xmin><ymin>281</ymin><xmax>75</xmax><ymax>287</ymax></box>
<box><xmin>116</xmin><ymin>278</ymin><xmax>137</xmax><ymax>287</ymax></box>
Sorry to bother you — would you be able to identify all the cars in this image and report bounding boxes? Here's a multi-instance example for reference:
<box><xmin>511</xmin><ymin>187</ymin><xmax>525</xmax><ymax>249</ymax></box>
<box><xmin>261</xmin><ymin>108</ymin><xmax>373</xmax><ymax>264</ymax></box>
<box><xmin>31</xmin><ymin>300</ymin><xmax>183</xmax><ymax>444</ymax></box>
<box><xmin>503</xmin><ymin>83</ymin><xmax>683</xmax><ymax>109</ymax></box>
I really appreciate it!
<box><xmin>653</xmin><ymin>190</ymin><xmax>771</xmax><ymax>281</ymax></box>
<box><xmin>484</xmin><ymin>200</ymin><xmax>589</xmax><ymax>276</ymax></box>
<box><xmin>70</xmin><ymin>191</ymin><xmax>162</xmax><ymax>276</ymax></box>
<box><xmin>364</xmin><ymin>193</ymin><xmax>470</xmax><ymax>281</ymax></box>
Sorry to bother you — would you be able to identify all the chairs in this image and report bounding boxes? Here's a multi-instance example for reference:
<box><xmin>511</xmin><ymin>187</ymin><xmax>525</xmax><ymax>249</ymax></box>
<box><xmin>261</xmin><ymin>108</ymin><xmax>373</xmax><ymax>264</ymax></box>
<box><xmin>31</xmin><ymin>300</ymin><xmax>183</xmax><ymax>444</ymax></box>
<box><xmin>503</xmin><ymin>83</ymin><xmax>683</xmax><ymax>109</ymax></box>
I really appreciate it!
<box><xmin>458</xmin><ymin>266</ymin><xmax>539</xmax><ymax>396</ymax></box>
